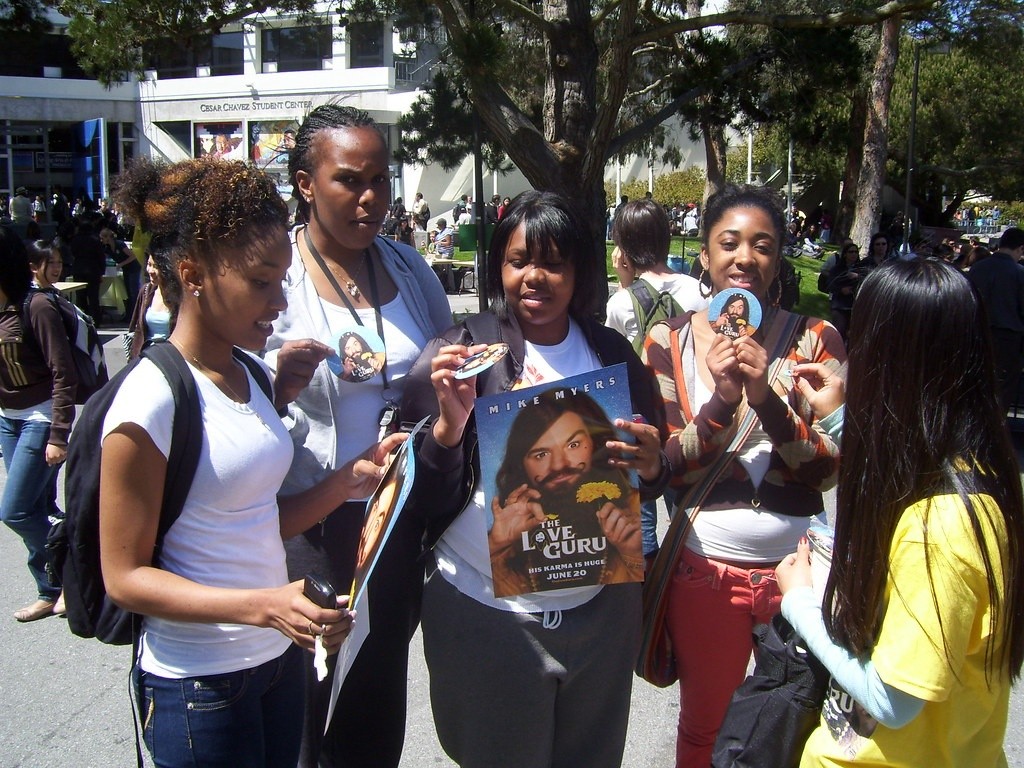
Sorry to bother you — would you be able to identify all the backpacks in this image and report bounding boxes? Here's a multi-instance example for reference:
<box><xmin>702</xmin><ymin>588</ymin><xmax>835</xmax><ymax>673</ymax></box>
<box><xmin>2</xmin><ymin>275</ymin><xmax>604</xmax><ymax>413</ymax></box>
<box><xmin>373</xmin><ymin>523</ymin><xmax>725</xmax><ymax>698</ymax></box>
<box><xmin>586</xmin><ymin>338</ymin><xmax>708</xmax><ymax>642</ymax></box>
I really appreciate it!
<box><xmin>453</xmin><ymin>204</ymin><xmax>459</xmax><ymax>217</ymax></box>
<box><xmin>625</xmin><ymin>277</ymin><xmax>686</xmax><ymax>364</ymax></box>
<box><xmin>23</xmin><ymin>287</ymin><xmax>109</xmax><ymax>404</ymax></box>
<box><xmin>45</xmin><ymin>338</ymin><xmax>273</xmax><ymax>768</ymax></box>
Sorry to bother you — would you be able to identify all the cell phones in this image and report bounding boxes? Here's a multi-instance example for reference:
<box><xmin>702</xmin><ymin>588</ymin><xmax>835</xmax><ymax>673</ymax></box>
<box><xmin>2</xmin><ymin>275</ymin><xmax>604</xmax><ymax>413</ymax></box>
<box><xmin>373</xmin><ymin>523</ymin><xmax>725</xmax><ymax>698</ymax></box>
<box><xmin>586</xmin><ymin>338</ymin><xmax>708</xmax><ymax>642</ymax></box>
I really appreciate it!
<box><xmin>303</xmin><ymin>573</ymin><xmax>337</xmax><ymax>609</ymax></box>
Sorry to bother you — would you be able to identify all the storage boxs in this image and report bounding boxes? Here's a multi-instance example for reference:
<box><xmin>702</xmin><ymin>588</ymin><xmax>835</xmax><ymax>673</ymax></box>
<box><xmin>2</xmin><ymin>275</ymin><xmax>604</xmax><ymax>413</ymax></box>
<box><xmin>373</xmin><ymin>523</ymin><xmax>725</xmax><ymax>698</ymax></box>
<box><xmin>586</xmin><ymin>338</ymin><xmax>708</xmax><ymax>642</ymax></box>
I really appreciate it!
<box><xmin>427</xmin><ymin>253</ymin><xmax>442</xmax><ymax>260</ymax></box>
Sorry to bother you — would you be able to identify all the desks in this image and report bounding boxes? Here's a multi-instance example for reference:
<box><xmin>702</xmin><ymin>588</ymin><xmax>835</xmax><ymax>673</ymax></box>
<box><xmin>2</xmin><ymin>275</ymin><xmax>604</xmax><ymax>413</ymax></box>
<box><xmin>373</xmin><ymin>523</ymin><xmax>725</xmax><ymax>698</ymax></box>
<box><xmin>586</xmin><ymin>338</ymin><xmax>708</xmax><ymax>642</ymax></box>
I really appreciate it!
<box><xmin>427</xmin><ymin>259</ymin><xmax>460</xmax><ymax>288</ymax></box>
<box><xmin>452</xmin><ymin>261</ymin><xmax>478</xmax><ymax>297</ymax></box>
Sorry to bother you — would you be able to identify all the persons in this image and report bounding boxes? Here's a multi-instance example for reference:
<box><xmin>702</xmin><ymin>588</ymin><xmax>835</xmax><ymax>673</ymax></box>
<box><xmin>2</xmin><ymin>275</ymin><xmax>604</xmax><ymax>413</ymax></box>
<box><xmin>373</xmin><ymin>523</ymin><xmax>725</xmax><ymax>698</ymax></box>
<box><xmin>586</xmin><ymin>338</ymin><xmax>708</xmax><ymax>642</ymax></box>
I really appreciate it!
<box><xmin>640</xmin><ymin>188</ymin><xmax>847</xmax><ymax>768</ymax></box>
<box><xmin>270</xmin><ymin>127</ymin><xmax>300</xmax><ymax>164</ymax></box>
<box><xmin>709</xmin><ymin>293</ymin><xmax>756</xmax><ymax>341</ymax></box>
<box><xmin>397</xmin><ymin>188</ymin><xmax>682</xmax><ymax>768</ymax></box>
<box><xmin>211</xmin><ymin>129</ymin><xmax>233</xmax><ymax>161</ymax></box>
<box><xmin>459</xmin><ymin>343</ymin><xmax>509</xmax><ymax>372</ymax></box>
<box><xmin>775</xmin><ymin>258</ymin><xmax>1024</xmax><ymax>768</ymax></box>
<box><xmin>261</xmin><ymin>103</ymin><xmax>456</xmax><ymax>768</ymax></box>
<box><xmin>486</xmin><ymin>388</ymin><xmax>644</xmax><ymax>598</ymax></box>
<box><xmin>377</xmin><ymin>192</ymin><xmax>1024</xmax><ymax>581</ymax></box>
<box><xmin>337</xmin><ymin>330</ymin><xmax>384</xmax><ymax>383</ymax></box>
<box><xmin>95</xmin><ymin>165</ymin><xmax>410</xmax><ymax>768</ymax></box>
<box><xmin>0</xmin><ymin>185</ymin><xmax>172</xmax><ymax>622</ymax></box>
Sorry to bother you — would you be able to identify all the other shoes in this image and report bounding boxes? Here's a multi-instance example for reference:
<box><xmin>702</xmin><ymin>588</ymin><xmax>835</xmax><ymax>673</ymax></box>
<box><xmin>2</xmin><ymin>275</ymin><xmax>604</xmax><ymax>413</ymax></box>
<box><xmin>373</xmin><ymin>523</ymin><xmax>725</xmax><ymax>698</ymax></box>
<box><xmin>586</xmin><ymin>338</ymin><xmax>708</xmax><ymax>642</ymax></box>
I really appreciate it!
<box><xmin>13</xmin><ymin>600</ymin><xmax>56</xmax><ymax>621</ymax></box>
<box><xmin>793</xmin><ymin>251</ymin><xmax>802</xmax><ymax>258</ymax></box>
<box><xmin>812</xmin><ymin>253</ymin><xmax>822</xmax><ymax>259</ymax></box>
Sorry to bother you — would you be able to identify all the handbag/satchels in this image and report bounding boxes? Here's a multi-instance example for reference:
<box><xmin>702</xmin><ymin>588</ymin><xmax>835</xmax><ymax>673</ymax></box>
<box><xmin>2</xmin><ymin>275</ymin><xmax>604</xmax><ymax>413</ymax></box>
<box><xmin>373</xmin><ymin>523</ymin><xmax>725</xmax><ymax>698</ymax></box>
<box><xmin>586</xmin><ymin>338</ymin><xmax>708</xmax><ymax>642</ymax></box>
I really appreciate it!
<box><xmin>423</xmin><ymin>208</ymin><xmax>430</xmax><ymax>220</ymax></box>
<box><xmin>635</xmin><ymin>548</ymin><xmax>678</xmax><ymax>688</ymax></box>
<box><xmin>712</xmin><ymin>612</ymin><xmax>830</xmax><ymax>768</ymax></box>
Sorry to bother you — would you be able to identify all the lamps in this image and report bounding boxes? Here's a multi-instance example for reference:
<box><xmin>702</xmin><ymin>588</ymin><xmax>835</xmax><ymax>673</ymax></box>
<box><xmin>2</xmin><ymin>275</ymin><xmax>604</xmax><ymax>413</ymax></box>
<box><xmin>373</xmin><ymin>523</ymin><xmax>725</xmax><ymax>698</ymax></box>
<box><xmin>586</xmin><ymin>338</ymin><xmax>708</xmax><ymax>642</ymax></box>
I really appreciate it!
<box><xmin>246</xmin><ymin>83</ymin><xmax>258</xmax><ymax>95</ymax></box>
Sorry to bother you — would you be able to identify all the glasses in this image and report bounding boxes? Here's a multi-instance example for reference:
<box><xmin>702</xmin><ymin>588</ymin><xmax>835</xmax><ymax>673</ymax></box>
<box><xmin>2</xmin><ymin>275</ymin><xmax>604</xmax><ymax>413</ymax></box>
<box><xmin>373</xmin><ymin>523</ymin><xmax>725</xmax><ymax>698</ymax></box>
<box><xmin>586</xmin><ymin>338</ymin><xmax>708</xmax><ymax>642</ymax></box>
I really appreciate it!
<box><xmin>875</xmin><ymin>243</ymin><xmax>887</xmax><ymax>246</ymax></box>
<box><xmin>849</xmin><ymin>251</ymin><xmax>859</xmax><ymax>253</ymax></box>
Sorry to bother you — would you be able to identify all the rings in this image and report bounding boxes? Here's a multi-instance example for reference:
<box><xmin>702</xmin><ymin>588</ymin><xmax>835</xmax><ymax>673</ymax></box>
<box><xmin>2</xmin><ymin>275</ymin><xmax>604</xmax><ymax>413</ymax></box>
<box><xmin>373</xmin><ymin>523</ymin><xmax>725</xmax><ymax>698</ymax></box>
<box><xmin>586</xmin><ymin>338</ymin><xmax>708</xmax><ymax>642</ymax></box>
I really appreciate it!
<box><xmin>308</xmin><ymin>620</ymin><xmax>317</xmax><ymax>636</ymax></box>
<box><xmin>503</xmin><ymin>498</ymin><xmax>517</xmax><ymax>506</ymax></box>
<box><xmin>322</xmin><ymin>624</ymin><xmax>327</xmax><ymax>636</ymax></box>
<box><xmin>622</xmin><ymin>512</ymin><xmax>633</xmax><ymax>521</ymax></box>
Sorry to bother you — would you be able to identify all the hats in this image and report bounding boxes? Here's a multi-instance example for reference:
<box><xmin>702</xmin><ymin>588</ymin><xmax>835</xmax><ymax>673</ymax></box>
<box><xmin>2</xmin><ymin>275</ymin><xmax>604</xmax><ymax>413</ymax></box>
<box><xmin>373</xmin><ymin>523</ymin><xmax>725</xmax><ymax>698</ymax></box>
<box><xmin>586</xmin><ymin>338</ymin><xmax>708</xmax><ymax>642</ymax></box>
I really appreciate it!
<box><xmin>16</xmin><ymin>187</ymin><xmax>28</xmax><ymax>194</ymax></box>
<box><xmin>436</xmin><ymin>218</ymin><xmax>446</xmax><ymax>224</ymax></box>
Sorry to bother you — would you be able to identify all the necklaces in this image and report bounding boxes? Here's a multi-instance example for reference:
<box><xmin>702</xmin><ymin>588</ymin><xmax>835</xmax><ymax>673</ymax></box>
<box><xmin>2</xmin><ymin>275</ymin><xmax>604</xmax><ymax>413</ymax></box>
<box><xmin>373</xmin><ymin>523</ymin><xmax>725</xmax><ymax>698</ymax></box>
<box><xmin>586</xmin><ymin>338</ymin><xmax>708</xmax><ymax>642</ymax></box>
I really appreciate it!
<box><xmin>319</xmin><ymin>253</ymin><xmax>368</xmax><ymax>298</ymax></box>
<box><xmin>170</xmin><ymin>334</ymin><xmax>270</xmax><ymax>432</ymax></box>
